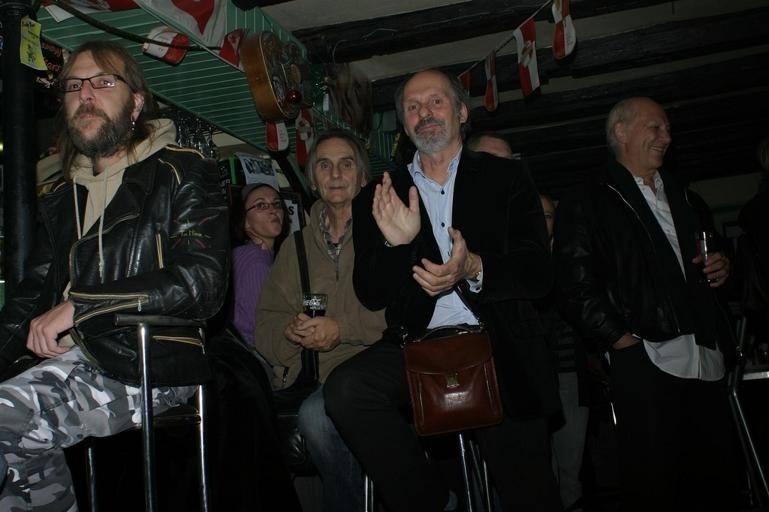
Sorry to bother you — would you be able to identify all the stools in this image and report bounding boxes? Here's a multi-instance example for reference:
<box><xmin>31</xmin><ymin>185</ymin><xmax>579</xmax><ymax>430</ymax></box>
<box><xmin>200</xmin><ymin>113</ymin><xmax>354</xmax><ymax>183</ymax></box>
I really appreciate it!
<box><xmin>363</xmin><ymin>430</ymin><xmax>492</xmax><ymax>510</ymax></box>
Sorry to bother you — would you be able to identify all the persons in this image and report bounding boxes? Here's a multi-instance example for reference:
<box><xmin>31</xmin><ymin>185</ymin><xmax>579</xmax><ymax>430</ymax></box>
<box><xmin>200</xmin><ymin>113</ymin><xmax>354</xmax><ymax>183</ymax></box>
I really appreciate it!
<box><xmin>540</xmin><ymin>195</ymin><xmax>590</xmax><ymax>511</ymax></box>
<box><xmin>255</xmin><ymin>128</ymin><xmax>388</xmax><ymax>512</ymax></box>
<box><xmin>0</xmin><ymin>38</ymin><xmax>232</xmax><ymax>512</ymax></box>
<box><xmin>467</xmin><ymin>135</ymin><xmax>520</xmax><ymax>161</ymax></box>
<box><xmin>552</xmin><ymin>96</ymin><xmax>735</xmax><ymax>510</ymax></box>
<box><xmin>322</xmin><ymin>68</ymin><xmax>567</xmax><ymax>511</ymax></box>
<box><xmin>223</xmin><ymin>184</ymin><xmax>292</xmax><ymax>361</ymax></box>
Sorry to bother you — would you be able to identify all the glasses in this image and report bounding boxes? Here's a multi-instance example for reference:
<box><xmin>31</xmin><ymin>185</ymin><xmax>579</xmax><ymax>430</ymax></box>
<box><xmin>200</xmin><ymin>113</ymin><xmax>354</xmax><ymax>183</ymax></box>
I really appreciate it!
<box><xmin>57</xmin><ymin>71</ymin><xmax>139</xmax><ymax>94</ymax></box>
<box><xmin>244</xmin><ymin>201</ymin><xmax>284</xmax><ymax>213</ymax></box>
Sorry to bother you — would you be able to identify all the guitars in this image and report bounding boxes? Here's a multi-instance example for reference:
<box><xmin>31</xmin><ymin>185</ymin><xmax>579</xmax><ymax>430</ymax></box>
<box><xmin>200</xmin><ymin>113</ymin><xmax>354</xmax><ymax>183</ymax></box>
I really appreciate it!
<box><xmin>242</xmin><ymin>30</ymin><xmax>332</xmax><ymax>121</ymax></box>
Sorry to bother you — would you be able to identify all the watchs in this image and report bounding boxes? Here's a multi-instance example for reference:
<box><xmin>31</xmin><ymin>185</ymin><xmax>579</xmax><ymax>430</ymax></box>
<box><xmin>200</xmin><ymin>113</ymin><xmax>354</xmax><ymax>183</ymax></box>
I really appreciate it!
<box><xmin>470</xmin><ymin>271</ymin><xmax>481</xmax><ymax>282</ymax></box>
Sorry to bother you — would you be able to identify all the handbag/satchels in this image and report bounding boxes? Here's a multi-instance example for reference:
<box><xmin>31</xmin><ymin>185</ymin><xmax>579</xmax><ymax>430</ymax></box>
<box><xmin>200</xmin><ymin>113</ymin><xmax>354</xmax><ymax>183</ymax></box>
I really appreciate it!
<box><xmin>272</xmin><ymin>382</ymin><xmax>322</xmax><ymax>477</ymax></box>
<box><xmin>404</xmin><ymin>330</ymin><xmax>505</xmax><ymax>437</ymax></box>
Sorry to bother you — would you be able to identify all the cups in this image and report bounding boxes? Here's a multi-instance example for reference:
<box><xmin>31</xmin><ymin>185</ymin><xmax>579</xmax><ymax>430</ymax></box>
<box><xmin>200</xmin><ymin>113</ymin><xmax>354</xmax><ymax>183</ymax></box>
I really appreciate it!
<box><xmin>302</xmin><ymin>292</ymin><xmax>330</xmax><ymax>316</ymax></box>
<box><xmin>698</xmin><ymin>226</ymin><xmax>721</xmax><ymax>284</ymax></box>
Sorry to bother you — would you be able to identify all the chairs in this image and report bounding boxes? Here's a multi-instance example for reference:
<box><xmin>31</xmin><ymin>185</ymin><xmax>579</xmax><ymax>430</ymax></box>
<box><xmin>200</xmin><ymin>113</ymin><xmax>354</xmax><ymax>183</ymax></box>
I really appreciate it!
<box><xmin>80</xmin><ymin>313</ymin><xmax>217</xmax><ymax>511</ymax></box>
